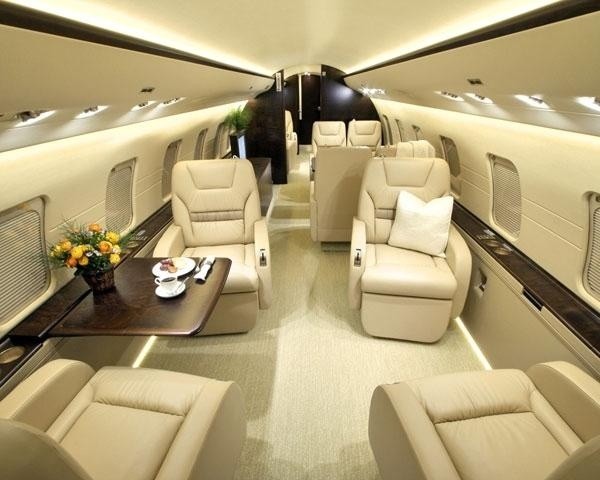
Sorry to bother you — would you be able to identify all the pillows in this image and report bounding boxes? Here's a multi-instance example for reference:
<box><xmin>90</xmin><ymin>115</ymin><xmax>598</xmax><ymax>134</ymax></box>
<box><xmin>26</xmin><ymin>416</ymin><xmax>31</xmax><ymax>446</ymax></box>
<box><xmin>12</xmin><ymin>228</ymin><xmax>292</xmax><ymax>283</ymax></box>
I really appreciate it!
<box><xmin>385</xmin><ymin>191</ymin><xmax>457</xmax><ymax>259</ymax></box>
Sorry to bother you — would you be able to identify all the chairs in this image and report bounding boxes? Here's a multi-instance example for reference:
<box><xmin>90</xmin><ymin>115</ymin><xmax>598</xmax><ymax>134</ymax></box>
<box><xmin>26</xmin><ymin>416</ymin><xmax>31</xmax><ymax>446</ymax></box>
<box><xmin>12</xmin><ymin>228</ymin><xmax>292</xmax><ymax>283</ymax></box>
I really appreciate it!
<box><xmin>152</xmin><ymin>158</ymin><xmax>273</xmax><ymax>337</ymax></box>
<box><xmin>0</xmin><ymin>358</ymin><xmax>248</xmax><ymax>480</ymax></box>
<box><xmin>368</xmin><ymin>360</ymin><xmax>600</xmax><ymax>480</ymax></box>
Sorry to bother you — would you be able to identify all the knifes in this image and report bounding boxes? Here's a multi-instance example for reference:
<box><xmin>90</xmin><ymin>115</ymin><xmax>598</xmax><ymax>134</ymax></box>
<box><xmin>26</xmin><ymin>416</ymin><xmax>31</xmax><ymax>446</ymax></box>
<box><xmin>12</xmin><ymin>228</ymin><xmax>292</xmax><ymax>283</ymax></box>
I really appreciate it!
<box><xmin>169</xmin><ymin>277</ymin><xmax>189</xmax><ymax>295</ymax></box>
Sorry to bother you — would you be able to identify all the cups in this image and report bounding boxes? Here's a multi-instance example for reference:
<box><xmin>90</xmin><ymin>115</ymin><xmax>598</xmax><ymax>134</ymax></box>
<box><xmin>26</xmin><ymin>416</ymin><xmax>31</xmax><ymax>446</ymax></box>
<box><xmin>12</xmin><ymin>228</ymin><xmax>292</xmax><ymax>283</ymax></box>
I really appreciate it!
<box><xmin>154</xmin><ymin>276</ymin><xmax>179</xmax><ymax>292</ymax></box>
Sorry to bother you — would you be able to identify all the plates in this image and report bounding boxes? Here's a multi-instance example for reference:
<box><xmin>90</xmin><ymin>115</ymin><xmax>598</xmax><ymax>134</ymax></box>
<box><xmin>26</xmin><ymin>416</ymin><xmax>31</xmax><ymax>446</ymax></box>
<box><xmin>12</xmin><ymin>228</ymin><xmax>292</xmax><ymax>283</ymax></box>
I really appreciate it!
<box><xmin>154</xmin><ymin>282</ymin><xmax>186</xmax><ymax>299</ymax></box>
<box><xmin>151</xmin><ymin>256</ymin><xmax>196</xmax><ymax>278</ymax></box>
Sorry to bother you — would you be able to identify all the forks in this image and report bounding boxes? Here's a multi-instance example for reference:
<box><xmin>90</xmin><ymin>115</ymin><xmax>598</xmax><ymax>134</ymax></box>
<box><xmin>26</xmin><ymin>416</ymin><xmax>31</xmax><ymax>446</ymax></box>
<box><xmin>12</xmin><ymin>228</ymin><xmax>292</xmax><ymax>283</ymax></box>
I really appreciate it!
<box><xmin>194</xmin><ymin>257</ymin><xmax>203</xmax><ymax>274</ymax></box>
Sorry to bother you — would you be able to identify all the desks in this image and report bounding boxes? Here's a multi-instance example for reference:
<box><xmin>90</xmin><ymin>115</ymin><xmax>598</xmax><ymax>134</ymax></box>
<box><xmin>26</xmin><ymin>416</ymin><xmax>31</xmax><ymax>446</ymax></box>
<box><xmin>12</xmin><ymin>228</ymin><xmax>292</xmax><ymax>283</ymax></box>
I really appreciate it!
<box><xmin>47</xmin><ymin>257</ymin><xmax>232</xmax><ymax>337</ymax></box>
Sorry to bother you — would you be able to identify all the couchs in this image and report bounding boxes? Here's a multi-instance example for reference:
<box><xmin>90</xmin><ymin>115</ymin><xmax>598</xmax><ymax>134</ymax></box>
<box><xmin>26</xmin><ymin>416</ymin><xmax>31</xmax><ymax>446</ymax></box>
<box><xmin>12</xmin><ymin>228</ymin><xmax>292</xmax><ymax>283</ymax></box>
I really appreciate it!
<box><xmin>308</xmin><ymin>119</ymin><xmax>346</xmax><ymax>179</ymax></box>
<box><xmin>308</xmin><ymin>145</ymin><xmax>372</xmax><ymax>252</ymax></box>
<box><xmin>346</xmin><ymin>154</ymin><xmax>474</xmax><ymax>344</ymax></box>
<box><xmin>347</xmin><ymin>119</ymin><xmax>383</xmax><ymax>149</ymax></box>
<box><xmin>373</xmin><ymin>141</ymin><xmax>435</xmax><ymax>159</ymax></box>
<box><xmin>285</xmin><ymin>111</ymin><xmax>301</xmax><ymax>172</ymax></box>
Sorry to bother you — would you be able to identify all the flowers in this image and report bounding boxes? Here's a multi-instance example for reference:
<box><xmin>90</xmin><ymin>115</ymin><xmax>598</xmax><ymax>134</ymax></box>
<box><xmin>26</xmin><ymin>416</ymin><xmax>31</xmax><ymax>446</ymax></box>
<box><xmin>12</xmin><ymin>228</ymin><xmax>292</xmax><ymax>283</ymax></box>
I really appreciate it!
<box><xmin>44</xmin><ymin>214</ymin><xmax>135</xmax><ymax>276</ymax></box>
